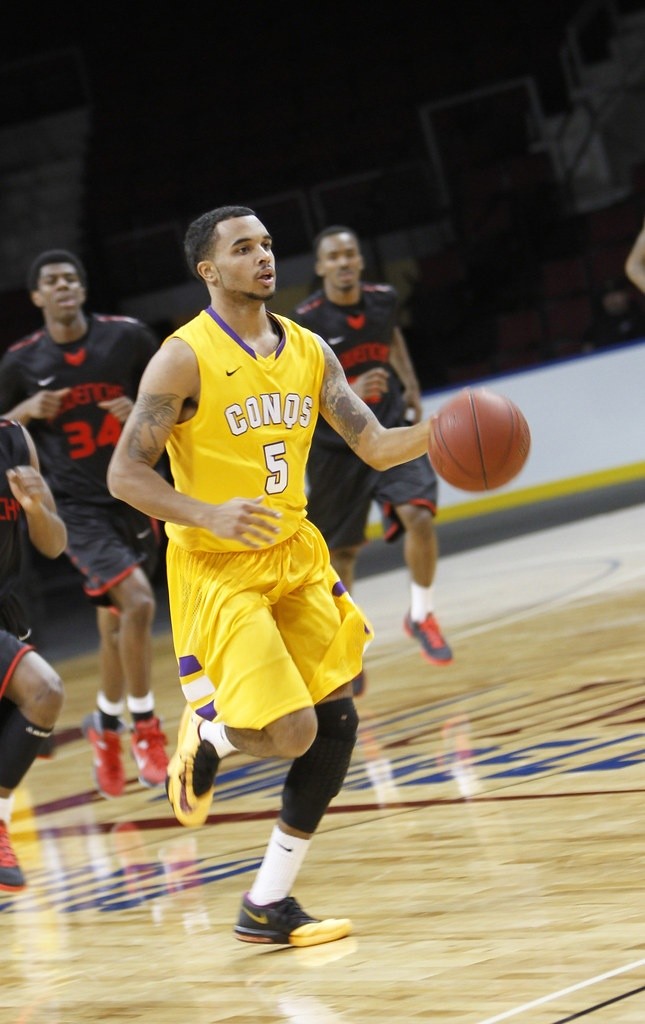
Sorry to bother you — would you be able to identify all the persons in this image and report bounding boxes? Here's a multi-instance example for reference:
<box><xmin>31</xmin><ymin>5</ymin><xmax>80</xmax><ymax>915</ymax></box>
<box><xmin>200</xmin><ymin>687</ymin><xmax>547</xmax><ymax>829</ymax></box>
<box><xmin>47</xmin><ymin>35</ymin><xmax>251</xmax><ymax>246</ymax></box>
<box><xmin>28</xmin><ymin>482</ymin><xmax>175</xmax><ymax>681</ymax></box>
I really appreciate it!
<box><xmin>289</xmin><ymin>224</ymin><xmax>456</xmax><ymax>665</ymax></box>
<box><xmin>0</xmin><ymin>417</ymin><xmax>67</xmax><ymax>897</ymax></box>
<box><xmin>107</xmin><ymin>208</ymin><xmax>438</xmax><ymax>946</ymax></box>
<box><xmin>1</xmin><ymin>249</ymin><xmax>170</xmax><ymax>799</ymax></box>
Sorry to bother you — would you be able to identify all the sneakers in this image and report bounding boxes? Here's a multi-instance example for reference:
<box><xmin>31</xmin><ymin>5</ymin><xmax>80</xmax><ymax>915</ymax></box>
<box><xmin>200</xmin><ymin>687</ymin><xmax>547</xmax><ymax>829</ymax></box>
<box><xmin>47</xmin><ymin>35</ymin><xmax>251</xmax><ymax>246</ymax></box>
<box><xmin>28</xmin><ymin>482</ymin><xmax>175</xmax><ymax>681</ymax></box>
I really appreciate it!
<box><xmin>130</xmin><ymin>718</ymin><xmax>170</xmax><ymax>789</ymax></box>
<box><xmin>234</xmin><ymin>892</ymin><xmax>352</xmax><ymax>947</ymax></box>
<box><xmin>0</xmin><ymin>818</ymin><xmax>26</xmax><ymax>892</ymax></box>
<box><xmin>82</xmin><ymin>711</ymin><xmax>126</xmax><ymax>801</ymax></box>
<box><xmin>403</xmin><ymin>608</ymin><xmax>452</xmax><ymax>666</ymax></box>
<box><xmin>352</xmin><ymin>669</ymin><xmax>365</xmax><ymax>697</ymax></box>
<box><xmin>165</xmin><ymin>704</ymin><xmax>220</xmax><ymax>828</ymax></box>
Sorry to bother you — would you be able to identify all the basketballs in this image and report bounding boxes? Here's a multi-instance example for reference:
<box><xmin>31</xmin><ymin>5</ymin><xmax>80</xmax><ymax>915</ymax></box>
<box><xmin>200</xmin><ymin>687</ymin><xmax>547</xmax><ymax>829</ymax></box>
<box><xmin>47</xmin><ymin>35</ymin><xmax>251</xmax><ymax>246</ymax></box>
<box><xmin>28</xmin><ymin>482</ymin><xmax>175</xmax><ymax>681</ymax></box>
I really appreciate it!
<box><xmin>424</xmin><ymin>388</ymin><xmax>532</xmax><ymax>493</ymax></box>
